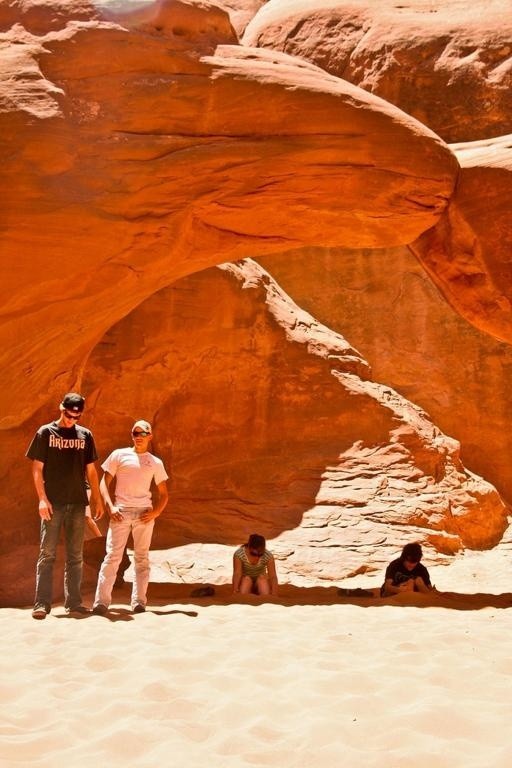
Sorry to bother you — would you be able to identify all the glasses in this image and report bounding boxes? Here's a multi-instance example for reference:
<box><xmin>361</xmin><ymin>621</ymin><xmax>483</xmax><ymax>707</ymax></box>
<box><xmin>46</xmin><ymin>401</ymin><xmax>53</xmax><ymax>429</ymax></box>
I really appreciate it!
<box><xmin>249</xmin><ymin>550</ymin><xmax>264</xmax><ymax>557</ymax></box>
<box><xmin>132</xmin><ymin>431</ymin><xmax>151</xmax><ymax>437</ymax></box>
<box><xmin>63</xmin><ymin>409</ymin><xmax>82</xmax><ymax>421</ymax></box>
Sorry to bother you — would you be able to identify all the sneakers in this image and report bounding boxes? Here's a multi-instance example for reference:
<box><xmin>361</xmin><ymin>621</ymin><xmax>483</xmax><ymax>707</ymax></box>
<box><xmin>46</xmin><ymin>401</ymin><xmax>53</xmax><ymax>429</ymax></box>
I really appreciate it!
<box><xmin>92</xmin><ymin>605</ymin><xmax>109</xmax><ymax>615</ymax></box>
<box><xmin>133</xmin><ymin>604</ymin><xmax>145</xmax><ymax>613</ymax></box>
<box><xmin>31</xmin><ymin>610</ymin><xmax>47</xmax><ymax>620</ymax></box>
<box><xmin>65</xmin><ymin>607</ymin><xmax>94</xmax><ymax>619</ymax></box>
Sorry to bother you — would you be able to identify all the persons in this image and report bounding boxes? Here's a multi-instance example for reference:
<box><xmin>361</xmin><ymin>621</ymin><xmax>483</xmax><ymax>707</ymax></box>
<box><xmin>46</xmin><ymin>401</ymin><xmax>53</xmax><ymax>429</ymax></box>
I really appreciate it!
<box><xmin>23</xmin><ymin>391</ymin><xmax>107</xmax><ymax>621</ymax></box>
<box><xmin>228</xmin><ymin>533</ymin><xmax>279</xmax><ymax>599</ymax></box>
<box><xmin>92</xmin><ymin>416</ymin><xmax>170</xmax><ymax>617</ymax></box>
<box><xmin>84</xmin><ymin>475</ymin><xmax>103</xmax><ymax>540</ymax></box>
<box><xmin>377</xmin><ymin>540</ymin><xmax>431</xmax><ymax>598</ymax></box>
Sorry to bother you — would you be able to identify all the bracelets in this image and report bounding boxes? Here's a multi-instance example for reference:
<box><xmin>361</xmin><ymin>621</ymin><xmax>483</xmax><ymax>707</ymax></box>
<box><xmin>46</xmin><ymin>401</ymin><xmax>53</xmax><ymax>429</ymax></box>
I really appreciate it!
<box><xmin>232</xmin><ymin>589</ymin><xmax>240</xmax><ymax>593</ymax></box>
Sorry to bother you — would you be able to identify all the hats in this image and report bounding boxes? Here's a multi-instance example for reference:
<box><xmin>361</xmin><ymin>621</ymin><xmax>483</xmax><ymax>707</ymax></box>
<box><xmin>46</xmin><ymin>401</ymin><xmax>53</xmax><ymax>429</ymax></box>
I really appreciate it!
<box><xmin>131</xmin><ymin>419</ymin><xmax>152</xmax><ymax>434</ymax></box>
<box><xmin>61</xmin><ymin>392</ymin><xmax>85</xmax><ymax>414</ymax></box>
